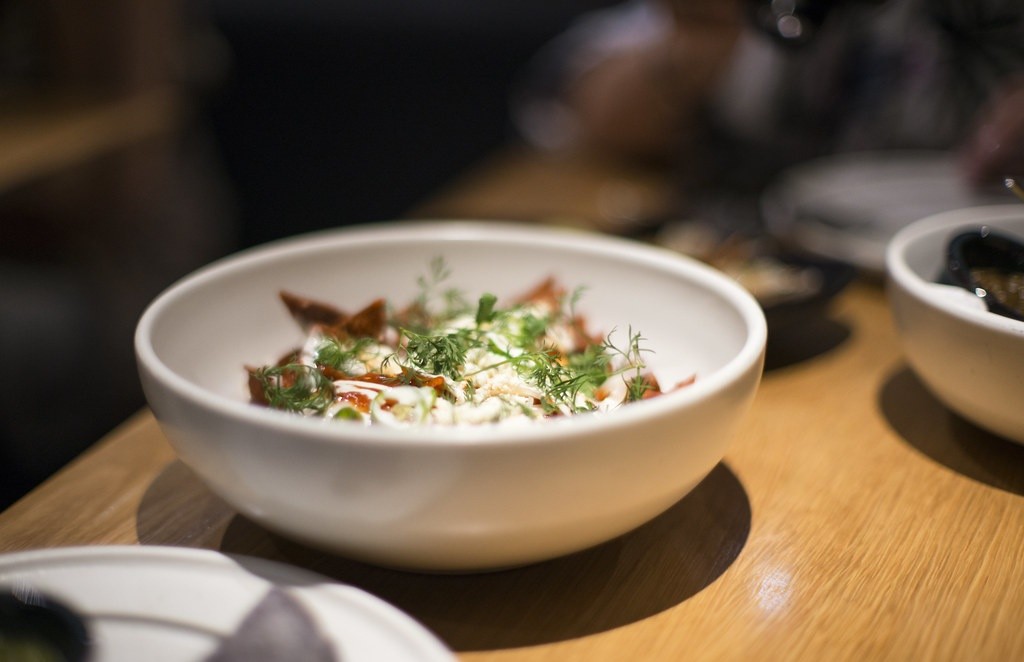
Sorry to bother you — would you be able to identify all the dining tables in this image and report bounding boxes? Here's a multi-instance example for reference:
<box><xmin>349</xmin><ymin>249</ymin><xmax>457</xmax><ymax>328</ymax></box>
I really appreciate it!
<box><xmin>0</xmin><ymin>150</ymin><xmax>1024</xmax><ymax>662</ymax></box>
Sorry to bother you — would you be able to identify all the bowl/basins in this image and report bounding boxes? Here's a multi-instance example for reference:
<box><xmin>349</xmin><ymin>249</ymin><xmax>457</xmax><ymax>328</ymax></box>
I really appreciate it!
<box><xmin>132</xmin><ymin>220</ymin><xmax>767</xmax><ymax>575</ymax></box>
<box><xmin>884</xmin><ymin>205</ymin><xmax>1024</xmax><ymax>450</ymax></box>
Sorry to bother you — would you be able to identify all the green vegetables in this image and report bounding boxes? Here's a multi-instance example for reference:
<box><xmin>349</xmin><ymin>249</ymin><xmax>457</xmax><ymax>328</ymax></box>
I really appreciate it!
<box><xmin>243</xmin><ymin>295</ymin><xmax>664</xmax><ymax>422</ymax></box>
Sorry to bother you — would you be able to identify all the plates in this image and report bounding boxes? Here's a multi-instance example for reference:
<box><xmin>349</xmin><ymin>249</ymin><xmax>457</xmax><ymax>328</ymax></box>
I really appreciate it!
<box><xmin>758</xmin><ymin>148</ymin><xmax>1017</xmax><ymax>274</ymax></box>
<box><xmin>619</xmin><ymin>207</ymin><xmax>851</xmax><ymax>336</ymax></box>
<box><xmin>0</xmin><ymin>544</ymin><xmax>462</xmax><ymax>662</ymax></box>
